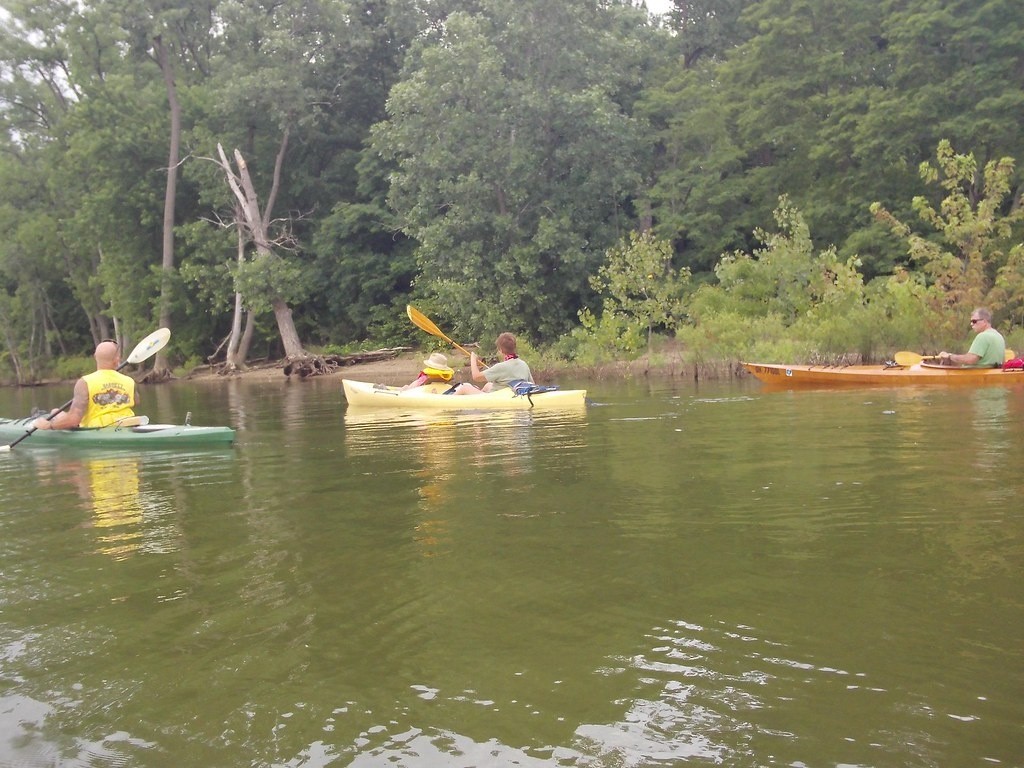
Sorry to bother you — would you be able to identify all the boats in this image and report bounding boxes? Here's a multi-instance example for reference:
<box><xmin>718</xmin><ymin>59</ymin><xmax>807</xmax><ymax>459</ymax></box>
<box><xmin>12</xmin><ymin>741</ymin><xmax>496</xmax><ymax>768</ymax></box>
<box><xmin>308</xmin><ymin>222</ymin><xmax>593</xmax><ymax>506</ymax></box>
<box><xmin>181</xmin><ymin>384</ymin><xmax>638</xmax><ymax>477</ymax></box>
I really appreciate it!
<box><xmin>739</xmin><ymin>358</ymin><xmax>1024</xmax><ymax>385</ymax></box>
<box><xmin>0</xmin><ymin>412</ymin><xmax>238</xmax><ymax>447</ymax></box>
<box><xmin>340</xmin><ymin>377</ymin><xmax>589</xmax><ymax>412</ymax></box>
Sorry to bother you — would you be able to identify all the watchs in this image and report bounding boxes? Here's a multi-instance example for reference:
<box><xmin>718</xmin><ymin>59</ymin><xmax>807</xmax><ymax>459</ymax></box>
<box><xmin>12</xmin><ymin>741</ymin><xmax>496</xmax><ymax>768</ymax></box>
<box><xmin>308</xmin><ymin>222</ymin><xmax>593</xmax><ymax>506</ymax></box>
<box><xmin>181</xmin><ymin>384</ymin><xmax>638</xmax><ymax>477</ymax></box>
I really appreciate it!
<box><xmin>948</xmin><ymin>353</ymin><xmax>952</xmax><ymax>359</ymax></box>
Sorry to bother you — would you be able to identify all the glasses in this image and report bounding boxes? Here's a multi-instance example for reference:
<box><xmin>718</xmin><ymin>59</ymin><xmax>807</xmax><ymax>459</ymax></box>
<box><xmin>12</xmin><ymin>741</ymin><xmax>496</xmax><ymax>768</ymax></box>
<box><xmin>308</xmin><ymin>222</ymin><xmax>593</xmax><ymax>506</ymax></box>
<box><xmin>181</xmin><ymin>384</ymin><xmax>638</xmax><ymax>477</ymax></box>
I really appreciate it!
<box><xmin>102</xmin><ymin>338</ymin><xmax>119</xmax><ymax>361</ymax></box>
<box><xmin>970</xmin><ymin>319</ymin><xmax>984</xmax><ymax>323</ymax></box>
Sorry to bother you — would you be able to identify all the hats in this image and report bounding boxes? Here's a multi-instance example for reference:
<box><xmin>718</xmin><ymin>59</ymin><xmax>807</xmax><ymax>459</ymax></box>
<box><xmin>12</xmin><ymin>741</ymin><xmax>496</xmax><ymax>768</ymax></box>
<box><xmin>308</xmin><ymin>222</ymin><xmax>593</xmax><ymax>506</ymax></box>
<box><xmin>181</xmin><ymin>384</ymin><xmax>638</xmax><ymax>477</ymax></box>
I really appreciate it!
<box><xmin>423</xmin><ymin>353</ymin><xmax>451</xmax><ymax>371</ymax></box>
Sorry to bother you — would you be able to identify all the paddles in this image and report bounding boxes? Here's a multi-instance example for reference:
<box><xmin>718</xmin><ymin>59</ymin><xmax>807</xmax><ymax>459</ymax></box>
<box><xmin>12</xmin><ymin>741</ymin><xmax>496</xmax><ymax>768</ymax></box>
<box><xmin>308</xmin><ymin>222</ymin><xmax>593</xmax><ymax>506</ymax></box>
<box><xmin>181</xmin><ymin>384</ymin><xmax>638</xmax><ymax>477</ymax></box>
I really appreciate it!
<box><xmin>894</xmin><ymin>348</ymin><xmax>1016</xmax><ymax>367</ymax></box>
<box><xmin>405</xmin><ymin>303</ymin><xmax>491</xmax><ymax>370</ymax></box>
<box><xmin>1</xmin><ymin>327</ymin><xmax>172</xmax><ymax>455</ymax></box>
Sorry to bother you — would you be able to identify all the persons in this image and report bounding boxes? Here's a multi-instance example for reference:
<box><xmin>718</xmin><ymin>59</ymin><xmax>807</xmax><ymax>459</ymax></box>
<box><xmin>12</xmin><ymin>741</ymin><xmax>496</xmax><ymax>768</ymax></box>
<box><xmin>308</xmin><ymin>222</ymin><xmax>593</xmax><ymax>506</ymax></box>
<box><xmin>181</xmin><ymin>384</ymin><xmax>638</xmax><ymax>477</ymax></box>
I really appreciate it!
<box><xmin>372</xmin><ymin>353</ymin><xmax>455</xmax><ymax>391</ymax></box>
<box><xmin>453</xmin><ymin>331</ymin><xmax>535</xmax><ymax>396</ymax></box>
<box><xmin>937</xmin><ymin>307</ymin><xmax>1005</xmax><ymax>368</ymax></box>
<box><xmin>31</xmin><ymin>339</ymin><xmax>141</xmax><ymax>431</ymax></box>
